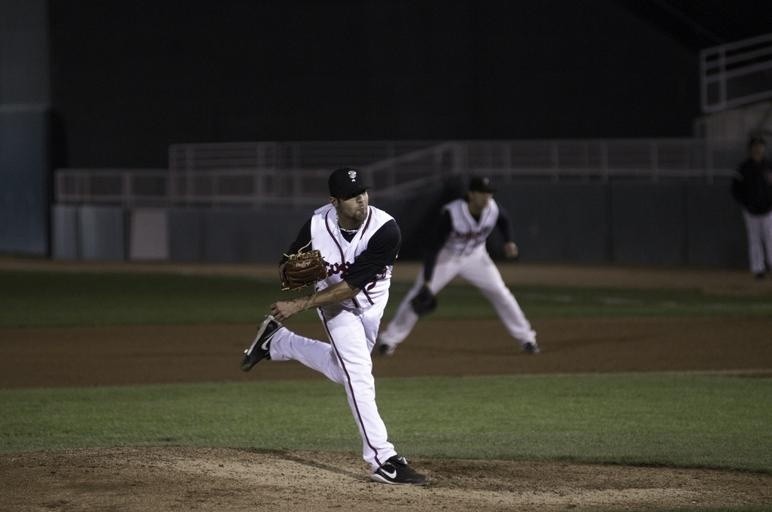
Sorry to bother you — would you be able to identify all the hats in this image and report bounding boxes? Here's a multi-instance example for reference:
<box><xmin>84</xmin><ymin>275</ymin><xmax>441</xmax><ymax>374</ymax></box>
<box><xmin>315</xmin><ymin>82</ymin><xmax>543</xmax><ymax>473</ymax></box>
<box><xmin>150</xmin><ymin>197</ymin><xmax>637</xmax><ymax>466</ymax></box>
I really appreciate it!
<box><xmin>468</xmin><ymin>175</ymin><xmax>499</xmax><ymax>194</ymax></box>
<box><xmin>327</xmin><ymin>165</ymin><xmax>378</xmax><ymax>200</ymax></box>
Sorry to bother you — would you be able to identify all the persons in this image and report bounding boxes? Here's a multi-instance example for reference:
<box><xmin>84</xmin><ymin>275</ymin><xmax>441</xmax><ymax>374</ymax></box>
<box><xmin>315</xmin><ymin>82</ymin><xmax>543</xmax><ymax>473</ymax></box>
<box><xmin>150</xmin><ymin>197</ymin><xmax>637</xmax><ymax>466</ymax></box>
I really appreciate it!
<box><xmin>728</xmin><ymin>137</ymin><xmax>771</xmax><ymax>279</ymax></box>
<box><xmin>240</xmin><ymin>167</ymin><xmax>431</xmax><ymax>487</ymax></box>
<box><xmin>378</xmin><ymin>170</ymin><xmax>543</xmax><ymax>359</ymax></box>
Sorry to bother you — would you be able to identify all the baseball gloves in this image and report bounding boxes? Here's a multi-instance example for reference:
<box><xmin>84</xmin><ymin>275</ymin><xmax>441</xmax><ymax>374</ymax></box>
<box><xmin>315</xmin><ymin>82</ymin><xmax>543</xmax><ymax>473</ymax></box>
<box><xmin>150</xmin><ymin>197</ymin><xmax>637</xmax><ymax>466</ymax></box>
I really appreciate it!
<box><xmin>410</xmin><ymin>286</ymin><xmax>437</xmax><ymax>316</ymax></box>
<box><xmin>282</xmin><ymin>250</ymin><xmax>328</xmax><ymax>290</ymax></box>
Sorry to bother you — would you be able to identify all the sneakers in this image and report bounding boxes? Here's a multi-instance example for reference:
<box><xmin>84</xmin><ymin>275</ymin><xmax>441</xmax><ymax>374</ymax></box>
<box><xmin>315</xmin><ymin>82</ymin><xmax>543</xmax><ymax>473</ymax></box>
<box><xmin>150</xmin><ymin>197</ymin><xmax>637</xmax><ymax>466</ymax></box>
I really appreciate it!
<box><xmin>523</xmin><ymin>340</ymin><xmax>541</xmax><ymax>354</ymax></box>
<box><xmin>239</xmin><ymin>313</ymin><xmax>284</xmax><ymax>372</ymax></box>
<box><xmin>755</xmin><ymin>272</ymin><xmax>766</xmax><ymax>280</ymax></box>
<box><xmin>379</xmin><ymin>343</ymin><xmax>394</xmax><ymax>356</ymax></box>
<box><xmin>369</xmin><ymin>453</ymin><xmax>429</xmax><ymax>486</ymax></box>
